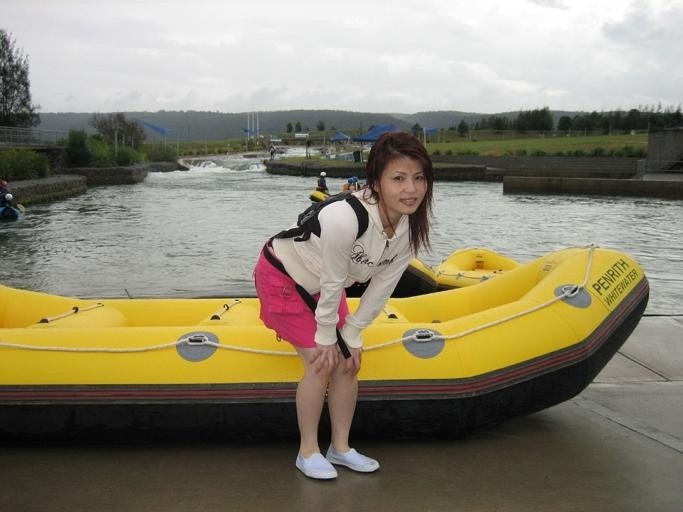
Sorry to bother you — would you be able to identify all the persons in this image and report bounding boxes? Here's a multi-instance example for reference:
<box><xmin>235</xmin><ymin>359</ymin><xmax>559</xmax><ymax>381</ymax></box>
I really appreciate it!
<box><xmin>253</xmin><ymin>132</ymin><xmax>434</xmax><ymax>481</ymax></box>
<box><xmin>343</xmin><ymin>176</ymin><xmax>369</xmax><ymax>192</ymax></box>
<box><xmin>269</xmin><ymin>145</ymin><xmax>276</xmax><ymax>159</ymax></box>
<box><xmin>318</xmin><ymin>171</ymin><xmax>328</xmax><ymax>193</ymax></box>
<box><xmin>0</xmin><ymin>179</ymin><xmax>14</xmax><ymax>208</ymax></box>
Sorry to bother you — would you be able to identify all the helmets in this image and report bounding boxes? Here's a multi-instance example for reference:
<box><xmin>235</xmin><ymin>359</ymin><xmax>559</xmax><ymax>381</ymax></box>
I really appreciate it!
<box><xmin>348</xmin><ymin>177</ymin><xmax>357</xmax><ymax>183</ymax></box>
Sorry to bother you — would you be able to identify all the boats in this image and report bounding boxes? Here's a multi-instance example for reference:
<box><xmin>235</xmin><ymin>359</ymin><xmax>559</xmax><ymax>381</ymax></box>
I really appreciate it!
<box><xmin>0</xmin><ymin>202</ymin><xmax>18</xmax><ymax>222</ymax></box>
<box><xmin>0</xmin><ymin>246</ymin><xmax>648</xmax><ymax>442</ymax></box>
<box><xmin>309</xmin><ymin>190</ymin><xmax>330</xmax><ymax>202</ymax></box>
<box><xmin>345</xmin><ymin>243</ymin><xmax>524</xmax><ymax>298</ymax></box>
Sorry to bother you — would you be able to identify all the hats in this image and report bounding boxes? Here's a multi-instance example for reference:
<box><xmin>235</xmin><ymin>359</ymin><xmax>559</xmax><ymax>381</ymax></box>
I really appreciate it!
<box><xmin>320</xmin><ymin>172</ymin><xmax>326</xmax><ymax>176</ymax></box>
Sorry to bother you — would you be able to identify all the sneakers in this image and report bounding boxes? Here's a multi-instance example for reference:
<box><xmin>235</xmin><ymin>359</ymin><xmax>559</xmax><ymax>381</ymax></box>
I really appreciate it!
<box><xmin>327</xmin><ymin>445</ymin><xmax>380</xmax><ymax>472</ymax></box>
<box><xmin>295</xmin><ymin>454</ymin><xmax>338</xmax><ymax>481</ymax></box>
